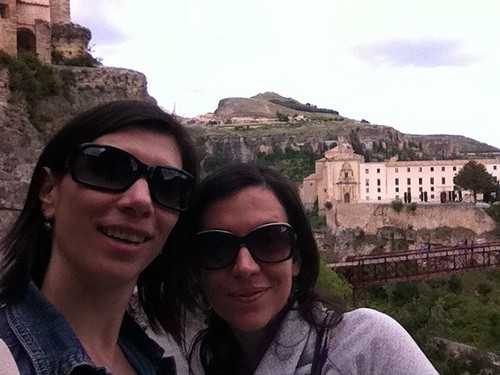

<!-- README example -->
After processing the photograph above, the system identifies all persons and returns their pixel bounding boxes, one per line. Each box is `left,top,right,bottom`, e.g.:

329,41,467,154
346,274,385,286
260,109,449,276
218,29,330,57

183,164,440,375
0,100,198,375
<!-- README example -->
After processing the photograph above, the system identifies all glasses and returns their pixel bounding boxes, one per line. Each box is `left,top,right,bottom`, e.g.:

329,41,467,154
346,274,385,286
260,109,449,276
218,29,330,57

189,222,299,271
61,143,198,212
